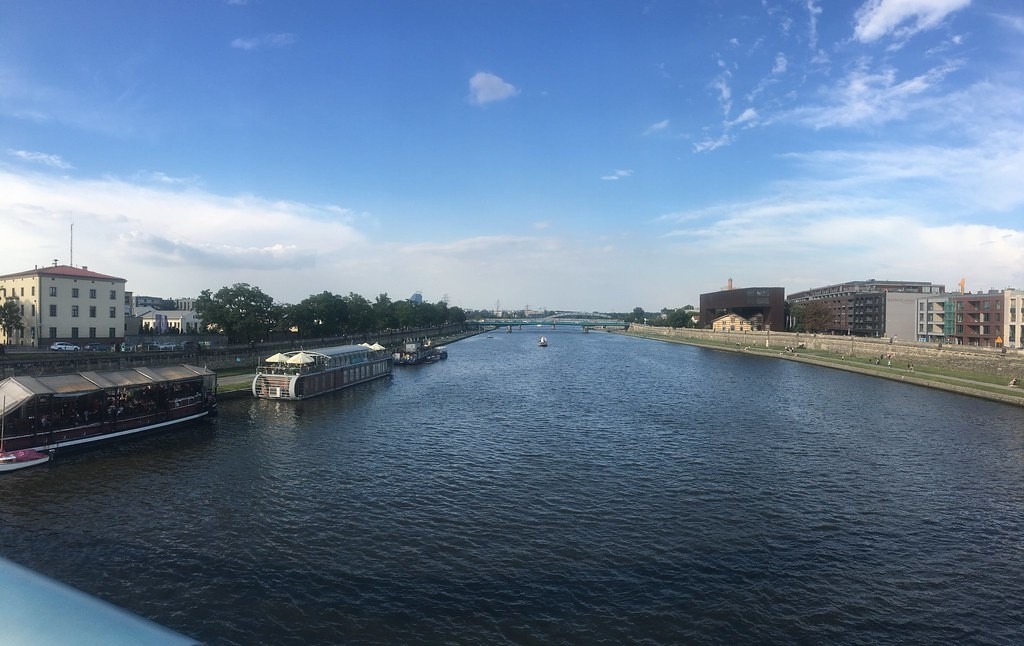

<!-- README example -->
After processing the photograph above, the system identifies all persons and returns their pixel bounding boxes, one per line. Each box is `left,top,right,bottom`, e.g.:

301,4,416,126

270,362,312,375
9,382,202,430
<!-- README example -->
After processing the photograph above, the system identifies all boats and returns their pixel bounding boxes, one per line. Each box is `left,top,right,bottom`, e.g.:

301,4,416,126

0,363,219,452
538,337,547,346
391,337,451,365
0,450,51,472
251,340,395,401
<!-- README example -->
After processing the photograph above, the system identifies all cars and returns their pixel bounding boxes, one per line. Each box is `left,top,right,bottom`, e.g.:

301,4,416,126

135,340,202,350
83,342,110,351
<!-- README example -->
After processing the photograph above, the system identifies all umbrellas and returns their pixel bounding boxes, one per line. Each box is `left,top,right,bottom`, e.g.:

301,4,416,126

362,342,371,348
369,342,385,354
286,352,314,373
265,353,289,373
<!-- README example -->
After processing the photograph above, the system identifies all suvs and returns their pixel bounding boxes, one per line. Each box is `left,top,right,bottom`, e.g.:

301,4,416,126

50,342,80,351
